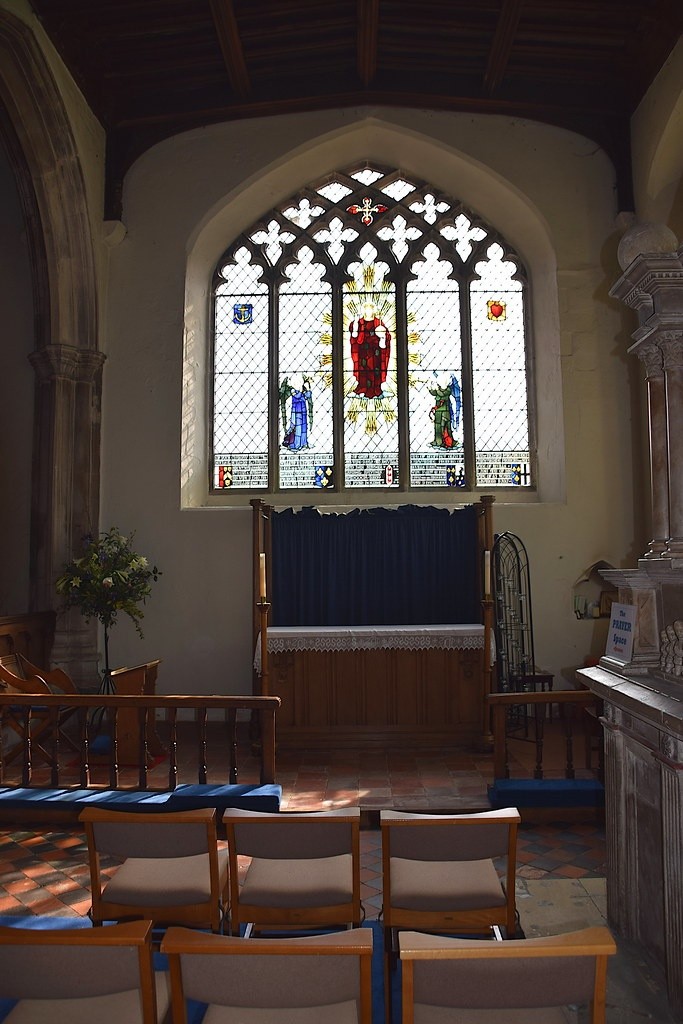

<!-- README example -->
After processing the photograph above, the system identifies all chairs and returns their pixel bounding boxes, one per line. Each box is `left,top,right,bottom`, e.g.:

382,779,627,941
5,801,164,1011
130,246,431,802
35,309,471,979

0,654,82,773
1,801,620,1024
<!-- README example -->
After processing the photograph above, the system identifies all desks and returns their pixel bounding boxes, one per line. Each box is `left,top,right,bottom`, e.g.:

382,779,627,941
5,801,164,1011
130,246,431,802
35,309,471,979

519,666,555,723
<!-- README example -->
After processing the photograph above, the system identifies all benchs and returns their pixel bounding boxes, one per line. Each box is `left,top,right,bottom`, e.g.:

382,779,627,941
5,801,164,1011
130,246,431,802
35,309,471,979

489,778,608,823
1,785,286,825
